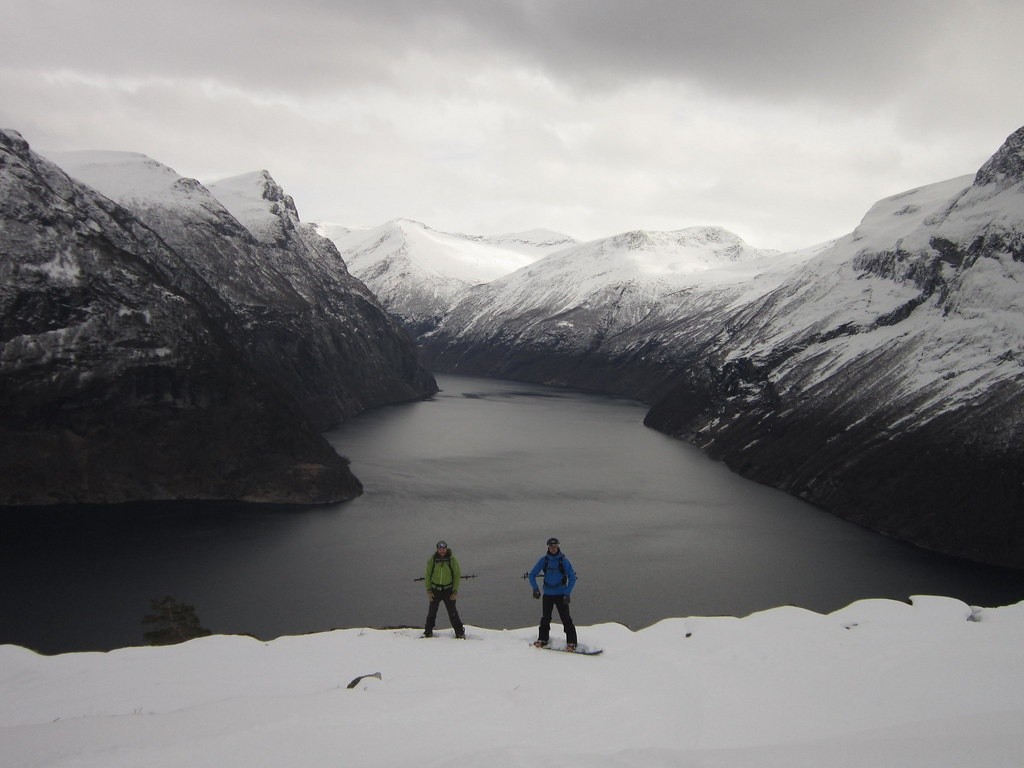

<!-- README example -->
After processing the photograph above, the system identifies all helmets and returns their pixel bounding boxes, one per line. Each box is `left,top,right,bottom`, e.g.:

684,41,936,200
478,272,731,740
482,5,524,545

547,538,560,545
436,540,447,548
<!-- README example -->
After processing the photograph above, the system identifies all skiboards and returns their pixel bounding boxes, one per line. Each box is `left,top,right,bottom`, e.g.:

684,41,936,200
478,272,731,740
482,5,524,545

414,632,480,640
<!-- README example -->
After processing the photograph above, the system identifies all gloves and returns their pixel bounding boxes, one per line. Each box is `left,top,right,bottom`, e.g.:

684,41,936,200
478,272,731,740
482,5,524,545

429,592,435,601
564,595,570,605
450,591,457,601
533,589,541,599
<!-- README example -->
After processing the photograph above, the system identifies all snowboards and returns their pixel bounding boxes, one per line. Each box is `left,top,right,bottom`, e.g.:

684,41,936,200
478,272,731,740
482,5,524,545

534,639,603,655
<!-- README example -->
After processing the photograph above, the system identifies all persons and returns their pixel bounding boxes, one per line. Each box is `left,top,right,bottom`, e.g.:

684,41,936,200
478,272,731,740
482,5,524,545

418,541,466,639
529,538,578,653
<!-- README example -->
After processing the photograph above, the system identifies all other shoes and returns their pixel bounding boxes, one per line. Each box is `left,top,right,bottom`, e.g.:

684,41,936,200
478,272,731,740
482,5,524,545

420,629,432,638
566,643,576,652
534,640,547,648
456,630,464,639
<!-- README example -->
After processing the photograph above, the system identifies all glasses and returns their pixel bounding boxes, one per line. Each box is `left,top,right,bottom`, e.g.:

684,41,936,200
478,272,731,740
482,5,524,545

549,545,558,549
437,543,446,548
